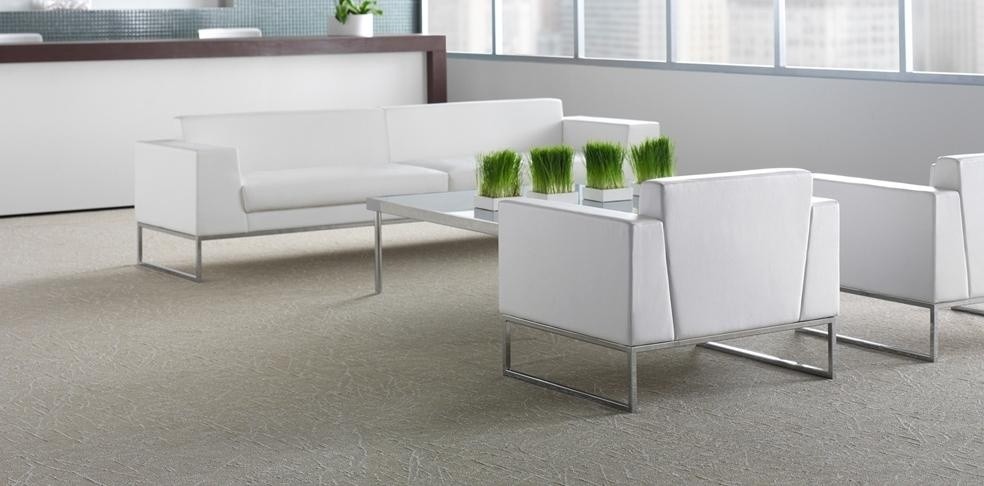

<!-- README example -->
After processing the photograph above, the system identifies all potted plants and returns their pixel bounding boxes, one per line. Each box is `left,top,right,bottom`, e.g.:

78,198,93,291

627,138,673,196
580,141,634,203
531,148,579,206
326,0,382,37
473,149,522,211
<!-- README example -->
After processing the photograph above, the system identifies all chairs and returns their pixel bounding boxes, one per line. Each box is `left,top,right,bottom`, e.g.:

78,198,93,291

198,26,264,38
1,31,44,42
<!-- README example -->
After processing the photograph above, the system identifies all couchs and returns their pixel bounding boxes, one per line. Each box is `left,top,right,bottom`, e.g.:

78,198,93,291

795,151,983,365
132,97,662,283
498,166,843,415
